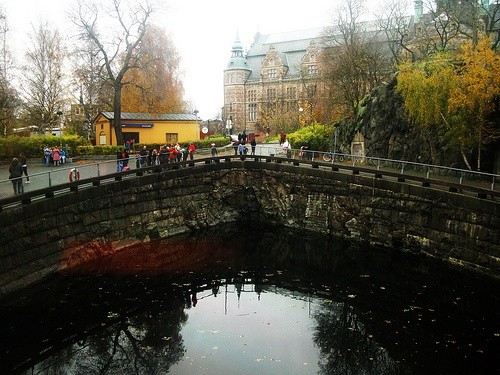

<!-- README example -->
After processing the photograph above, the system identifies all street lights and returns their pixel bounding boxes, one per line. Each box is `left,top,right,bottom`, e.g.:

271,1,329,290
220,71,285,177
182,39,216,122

299,108,304,129
333,122,339,162
56,111,63,147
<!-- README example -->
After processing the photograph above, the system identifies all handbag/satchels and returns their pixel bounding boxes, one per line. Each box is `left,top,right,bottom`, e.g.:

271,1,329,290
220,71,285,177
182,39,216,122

283,147,288,151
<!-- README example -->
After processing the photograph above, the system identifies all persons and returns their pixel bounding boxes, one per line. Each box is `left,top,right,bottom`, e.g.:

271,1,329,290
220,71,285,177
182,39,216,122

19,152,30,184
117,147,130,176
251,139,256,152
300,142,312,160
135,142,195,168
9,158,23,194
126,138,135,154
283,138,291,159
210,143,217,162
42,144,70,167
280,131,284,144
233,131,248,154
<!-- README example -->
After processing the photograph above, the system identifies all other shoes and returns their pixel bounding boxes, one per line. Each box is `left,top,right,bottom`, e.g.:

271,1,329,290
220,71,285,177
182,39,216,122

24,181,30,183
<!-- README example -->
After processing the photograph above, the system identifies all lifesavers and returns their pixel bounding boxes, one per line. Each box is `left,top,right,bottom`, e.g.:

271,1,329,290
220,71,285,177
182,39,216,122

69,168,80,182
